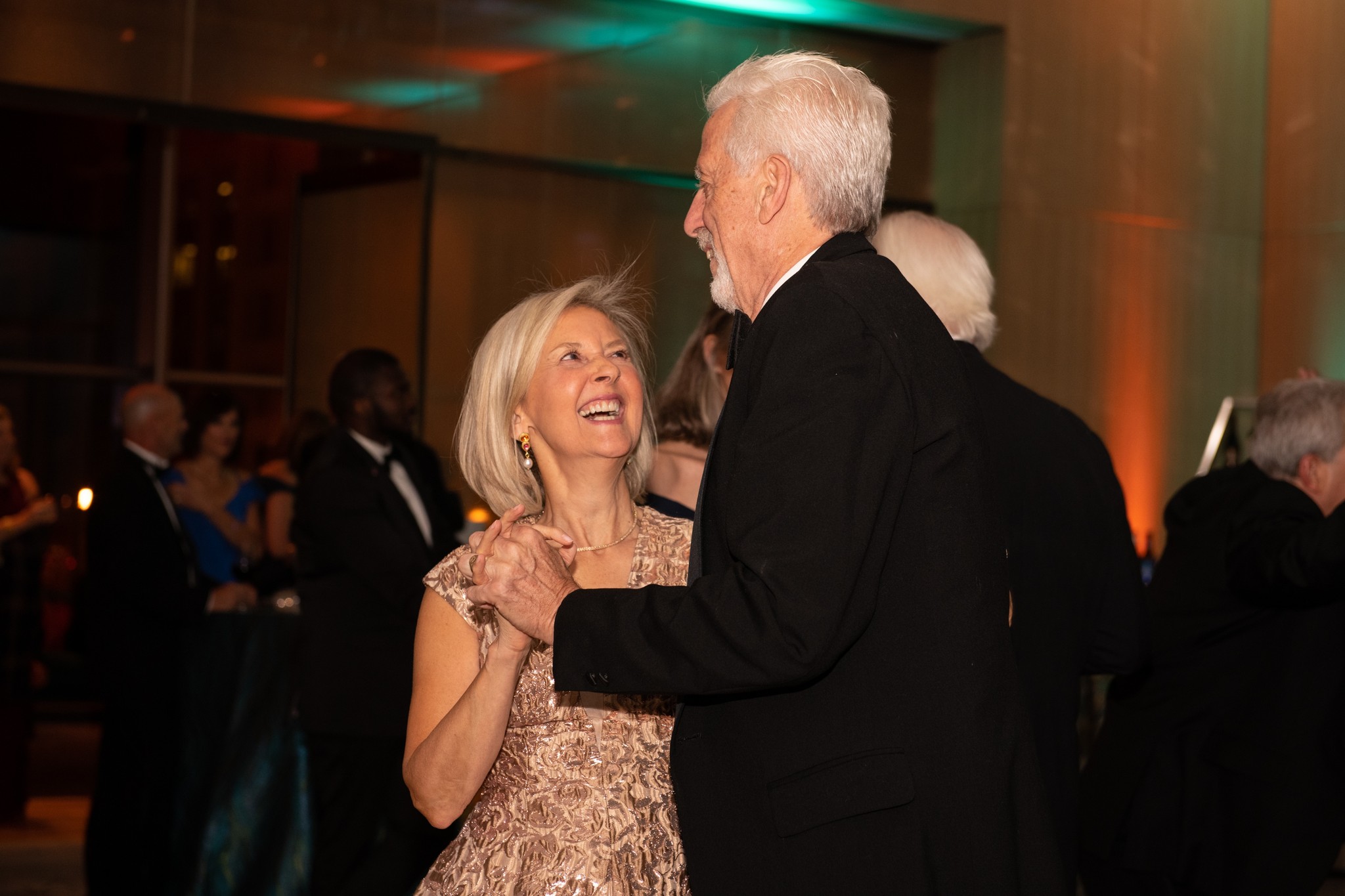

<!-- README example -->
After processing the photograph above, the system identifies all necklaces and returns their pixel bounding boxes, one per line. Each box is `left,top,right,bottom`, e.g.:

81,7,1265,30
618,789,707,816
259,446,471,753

528,508,638,556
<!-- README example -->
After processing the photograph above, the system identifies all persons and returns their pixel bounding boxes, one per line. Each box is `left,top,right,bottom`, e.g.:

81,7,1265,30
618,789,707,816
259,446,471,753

400,250,695,896
1148,372,1345,894
0,208,1149,896
461,43,1037,896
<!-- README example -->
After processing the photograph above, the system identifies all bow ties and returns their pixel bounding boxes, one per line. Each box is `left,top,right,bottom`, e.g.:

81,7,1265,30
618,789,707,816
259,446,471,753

726,308,750,370
382,448,399,475
